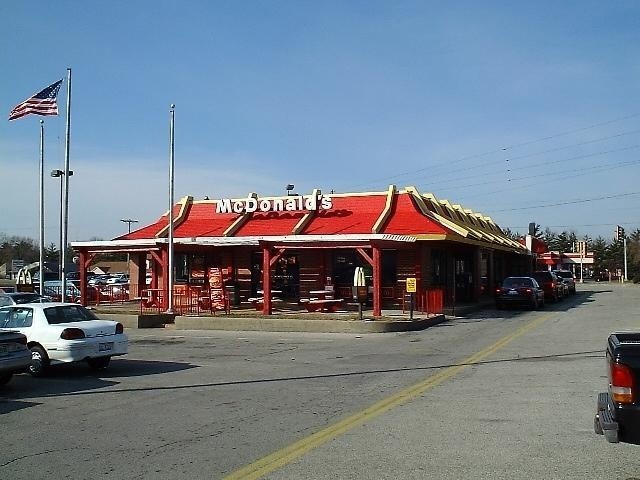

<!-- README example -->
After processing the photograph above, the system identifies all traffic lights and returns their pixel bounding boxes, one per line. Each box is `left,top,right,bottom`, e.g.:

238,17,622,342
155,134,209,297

574,241,589,253
617,225,624,241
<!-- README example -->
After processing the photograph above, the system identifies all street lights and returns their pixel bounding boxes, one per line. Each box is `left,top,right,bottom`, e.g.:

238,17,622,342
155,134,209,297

120,219,138,234
51,169,74,280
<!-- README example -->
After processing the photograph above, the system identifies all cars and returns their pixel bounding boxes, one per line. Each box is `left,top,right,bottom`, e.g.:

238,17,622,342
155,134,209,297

0,271,153,305
480,276,488,295
593,273,609,282
1,301,129,377
495,270,576,310
1,330,32,385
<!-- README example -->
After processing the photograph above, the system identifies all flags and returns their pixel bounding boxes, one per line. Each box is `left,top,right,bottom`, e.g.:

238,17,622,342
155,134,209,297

8,79,63,119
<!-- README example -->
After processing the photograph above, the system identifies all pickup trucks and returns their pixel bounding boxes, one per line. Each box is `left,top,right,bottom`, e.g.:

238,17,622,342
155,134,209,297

594,329,640,446
